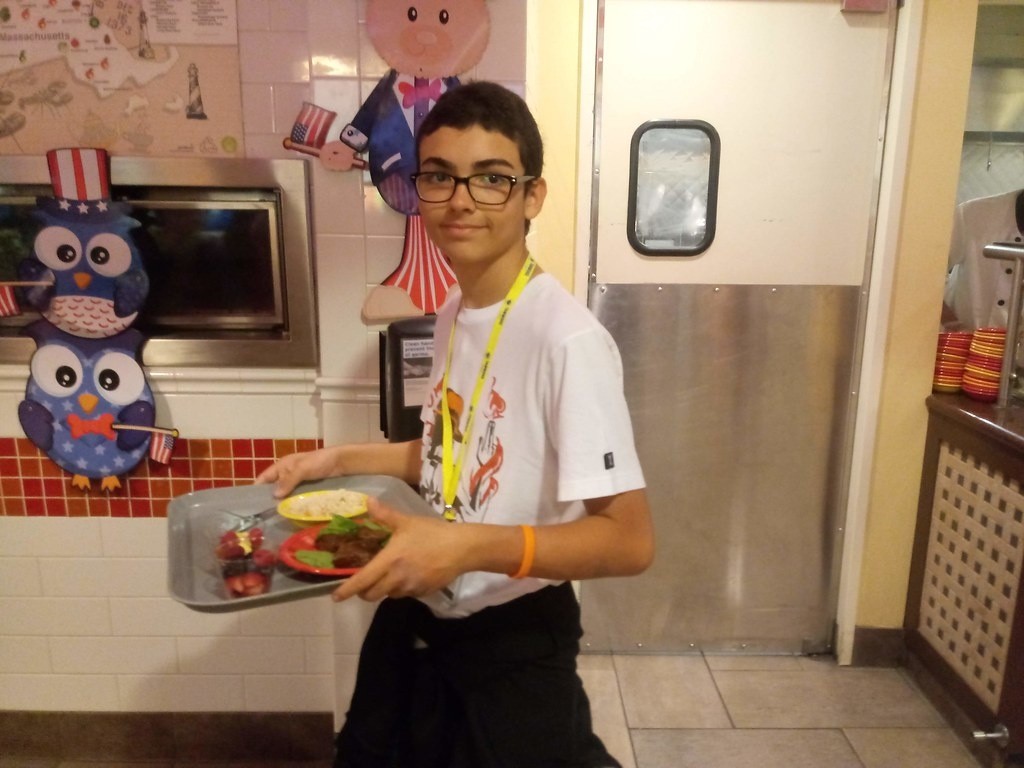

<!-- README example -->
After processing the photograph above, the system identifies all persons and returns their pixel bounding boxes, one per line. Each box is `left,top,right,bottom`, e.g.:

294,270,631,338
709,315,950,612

939,188,1024,374
254,79,655,768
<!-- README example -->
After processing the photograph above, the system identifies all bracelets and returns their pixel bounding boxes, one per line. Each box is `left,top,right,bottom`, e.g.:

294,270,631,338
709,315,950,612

510,524,536,580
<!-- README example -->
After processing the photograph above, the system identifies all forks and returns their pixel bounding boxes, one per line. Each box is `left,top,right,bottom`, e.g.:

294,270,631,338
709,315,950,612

218,508,278,522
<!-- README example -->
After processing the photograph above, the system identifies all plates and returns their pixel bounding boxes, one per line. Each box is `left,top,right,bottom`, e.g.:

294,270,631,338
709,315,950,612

278,519,391,575
934,326,1007,400
275,490,371,525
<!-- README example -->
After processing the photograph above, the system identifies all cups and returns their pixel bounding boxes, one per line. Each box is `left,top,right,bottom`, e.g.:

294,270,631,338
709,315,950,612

215,517,264,551
214,537,274,594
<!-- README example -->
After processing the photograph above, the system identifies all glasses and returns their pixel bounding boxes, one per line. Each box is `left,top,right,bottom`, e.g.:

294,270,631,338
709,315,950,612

409,171,536,207
